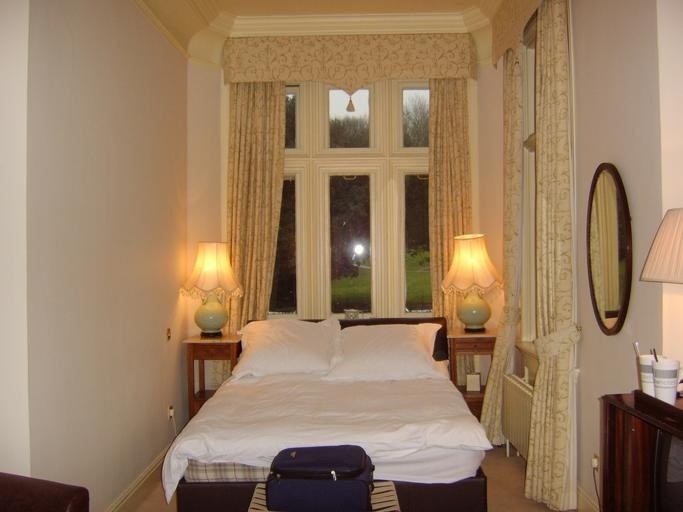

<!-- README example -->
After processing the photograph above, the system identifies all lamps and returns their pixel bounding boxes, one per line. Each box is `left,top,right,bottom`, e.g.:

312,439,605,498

441,233,498,332
640,207,683,284
180,240,244,337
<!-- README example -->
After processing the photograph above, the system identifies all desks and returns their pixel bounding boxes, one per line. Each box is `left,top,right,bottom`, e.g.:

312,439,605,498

597,390,683,511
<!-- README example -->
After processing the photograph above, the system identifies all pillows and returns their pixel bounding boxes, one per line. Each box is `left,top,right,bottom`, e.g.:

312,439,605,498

327,322,445,379
231,318,341,376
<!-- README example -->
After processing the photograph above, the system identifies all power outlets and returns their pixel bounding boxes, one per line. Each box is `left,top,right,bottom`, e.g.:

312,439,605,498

169,405,173,419
593,454,600,471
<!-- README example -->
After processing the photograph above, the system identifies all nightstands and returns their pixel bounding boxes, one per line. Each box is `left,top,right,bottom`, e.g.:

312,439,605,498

447,333,496,402
182,335,242,418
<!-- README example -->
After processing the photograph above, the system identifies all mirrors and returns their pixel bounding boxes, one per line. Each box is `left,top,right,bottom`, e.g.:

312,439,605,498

586,162,632,334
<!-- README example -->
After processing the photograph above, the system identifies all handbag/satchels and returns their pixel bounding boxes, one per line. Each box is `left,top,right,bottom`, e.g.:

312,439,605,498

266,445,374,512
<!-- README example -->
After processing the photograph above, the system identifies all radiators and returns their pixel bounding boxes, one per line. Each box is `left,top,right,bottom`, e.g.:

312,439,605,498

502,374,534,460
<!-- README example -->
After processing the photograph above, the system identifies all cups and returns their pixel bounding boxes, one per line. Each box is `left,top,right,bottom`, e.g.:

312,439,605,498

637,355,678,408
344,309,360,320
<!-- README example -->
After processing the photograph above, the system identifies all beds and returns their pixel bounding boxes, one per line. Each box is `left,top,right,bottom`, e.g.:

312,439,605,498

161,317,494,512
